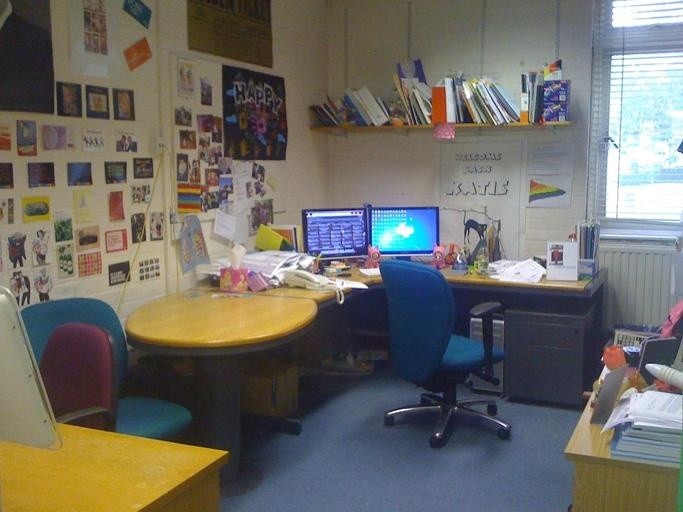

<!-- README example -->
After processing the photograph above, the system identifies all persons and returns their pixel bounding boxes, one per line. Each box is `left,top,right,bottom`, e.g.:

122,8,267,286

32,230,49,265
7,232,26,268
9,270,30,305
433,243,445,269
34,268,50,302
116,134,137,152
365,244,381,267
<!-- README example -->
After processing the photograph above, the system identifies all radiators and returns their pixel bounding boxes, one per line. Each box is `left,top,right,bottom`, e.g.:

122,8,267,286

596,244,679,339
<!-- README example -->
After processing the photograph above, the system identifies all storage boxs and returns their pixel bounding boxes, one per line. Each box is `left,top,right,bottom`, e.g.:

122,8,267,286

238,357,300,418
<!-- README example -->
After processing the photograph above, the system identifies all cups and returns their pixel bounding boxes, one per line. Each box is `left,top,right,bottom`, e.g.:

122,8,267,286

603,346,625,371
623,346,640,368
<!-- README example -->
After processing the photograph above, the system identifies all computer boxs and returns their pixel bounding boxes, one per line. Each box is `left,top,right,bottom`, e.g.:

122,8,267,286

470,315,504,396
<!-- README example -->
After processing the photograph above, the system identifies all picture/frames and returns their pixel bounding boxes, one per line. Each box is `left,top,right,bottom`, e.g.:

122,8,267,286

525,175,573,209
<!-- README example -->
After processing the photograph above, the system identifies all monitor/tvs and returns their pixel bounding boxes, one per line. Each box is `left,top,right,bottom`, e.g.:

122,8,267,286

302,207,369,264
369,206,440,261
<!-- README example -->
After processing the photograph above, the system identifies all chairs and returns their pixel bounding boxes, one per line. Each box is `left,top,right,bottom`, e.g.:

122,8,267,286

38,322,117,432
20,298,198,446
378,257,512,447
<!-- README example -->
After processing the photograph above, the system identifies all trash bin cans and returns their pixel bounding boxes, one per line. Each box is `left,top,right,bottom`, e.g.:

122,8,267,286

138,355,205,414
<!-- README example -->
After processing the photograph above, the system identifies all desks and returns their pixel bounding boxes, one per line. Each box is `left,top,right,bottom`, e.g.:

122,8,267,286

563,331,682,512
203,257,609,407
0,423,231,512
124,288,318,497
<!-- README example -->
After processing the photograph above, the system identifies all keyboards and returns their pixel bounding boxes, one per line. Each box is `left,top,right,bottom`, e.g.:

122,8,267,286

360,267,380,276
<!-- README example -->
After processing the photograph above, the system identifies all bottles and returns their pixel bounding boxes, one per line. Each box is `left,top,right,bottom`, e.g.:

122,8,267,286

444,243,466,271
476,240,489,278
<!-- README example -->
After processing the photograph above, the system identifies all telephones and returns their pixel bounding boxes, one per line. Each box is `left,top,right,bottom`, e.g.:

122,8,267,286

285,270,328,288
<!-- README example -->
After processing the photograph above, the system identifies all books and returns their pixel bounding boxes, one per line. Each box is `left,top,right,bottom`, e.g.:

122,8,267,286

309,71,538,127
610,390,683,462
613,330,653,347
